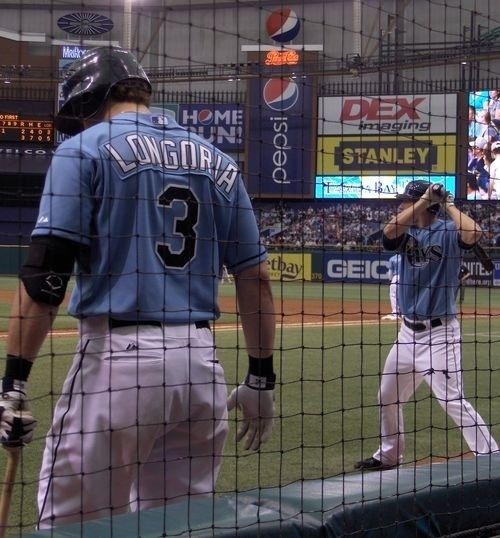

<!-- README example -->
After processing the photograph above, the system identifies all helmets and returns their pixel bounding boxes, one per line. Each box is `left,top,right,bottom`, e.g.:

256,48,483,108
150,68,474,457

54,45,152,136
395,180,440,215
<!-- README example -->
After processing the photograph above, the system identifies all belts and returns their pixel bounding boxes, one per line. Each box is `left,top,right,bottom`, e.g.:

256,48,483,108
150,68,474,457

404,317,442,331
109,318,210,330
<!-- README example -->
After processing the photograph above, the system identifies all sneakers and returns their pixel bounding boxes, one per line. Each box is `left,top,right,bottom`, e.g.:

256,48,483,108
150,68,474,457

354,457,382,469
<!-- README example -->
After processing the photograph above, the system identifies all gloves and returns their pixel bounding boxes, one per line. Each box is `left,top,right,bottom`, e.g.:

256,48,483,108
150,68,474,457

0,353,38,447
227,354,276,450
420,183,454,211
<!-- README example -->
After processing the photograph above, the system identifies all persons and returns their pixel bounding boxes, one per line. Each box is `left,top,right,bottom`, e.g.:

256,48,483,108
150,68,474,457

356,180,499,470
468,90,500,200
0,46,276,531
256,201,500,252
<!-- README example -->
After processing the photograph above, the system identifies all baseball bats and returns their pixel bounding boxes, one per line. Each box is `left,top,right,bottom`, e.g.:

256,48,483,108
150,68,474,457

432,184,495,273
1,400,26,537
